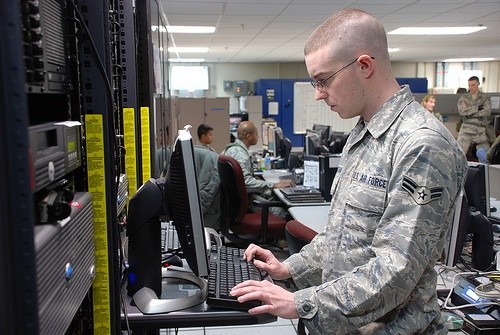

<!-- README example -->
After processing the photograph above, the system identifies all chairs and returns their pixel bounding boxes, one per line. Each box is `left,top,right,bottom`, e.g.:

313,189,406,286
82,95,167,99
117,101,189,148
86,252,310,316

217,156,290,252
285,220,319,335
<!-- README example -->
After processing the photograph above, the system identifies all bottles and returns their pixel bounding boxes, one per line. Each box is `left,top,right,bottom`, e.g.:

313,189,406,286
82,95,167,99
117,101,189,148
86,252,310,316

265,153,271,170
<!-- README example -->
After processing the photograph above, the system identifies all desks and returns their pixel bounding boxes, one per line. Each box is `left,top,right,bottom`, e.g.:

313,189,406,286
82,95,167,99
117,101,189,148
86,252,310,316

260,169,330,209
286,205,330,234
119,266,278,335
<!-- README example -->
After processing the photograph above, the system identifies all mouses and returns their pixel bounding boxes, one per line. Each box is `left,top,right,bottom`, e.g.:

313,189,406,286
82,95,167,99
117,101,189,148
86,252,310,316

160,254,183,267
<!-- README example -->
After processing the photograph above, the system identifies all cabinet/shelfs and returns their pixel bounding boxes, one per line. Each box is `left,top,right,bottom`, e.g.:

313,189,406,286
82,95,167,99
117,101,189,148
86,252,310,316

170,97,230,154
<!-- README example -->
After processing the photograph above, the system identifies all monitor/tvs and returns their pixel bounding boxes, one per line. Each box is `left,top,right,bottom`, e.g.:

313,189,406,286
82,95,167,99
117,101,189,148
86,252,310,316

304,123,331,157
445,186,495,271
302,155,327,194
125,131,210,315
463,161,490,218
260,122,290,163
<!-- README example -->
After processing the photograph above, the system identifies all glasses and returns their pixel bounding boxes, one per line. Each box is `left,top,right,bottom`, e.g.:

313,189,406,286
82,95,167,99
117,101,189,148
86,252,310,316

310,56,375,91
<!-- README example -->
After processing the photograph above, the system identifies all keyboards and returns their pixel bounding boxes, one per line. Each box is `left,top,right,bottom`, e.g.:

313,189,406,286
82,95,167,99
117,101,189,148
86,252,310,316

159,223,185,258
279,174,301,184
204,243,263,312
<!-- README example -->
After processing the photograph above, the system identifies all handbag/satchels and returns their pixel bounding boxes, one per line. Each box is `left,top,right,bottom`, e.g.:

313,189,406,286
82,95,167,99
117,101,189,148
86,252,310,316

485,124,497,146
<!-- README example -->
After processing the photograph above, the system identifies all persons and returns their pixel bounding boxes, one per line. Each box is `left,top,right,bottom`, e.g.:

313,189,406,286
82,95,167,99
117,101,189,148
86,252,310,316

194,124,216,152
457,76,492,164
230,9,470,335
421,95,443,123
220,121,296,218
